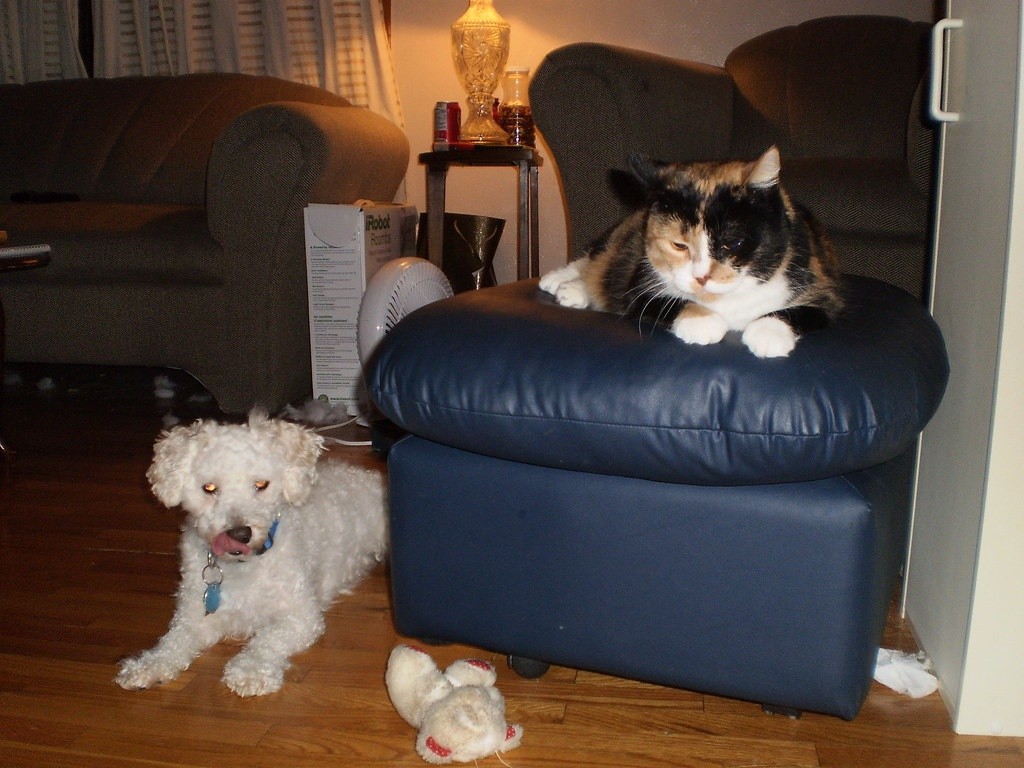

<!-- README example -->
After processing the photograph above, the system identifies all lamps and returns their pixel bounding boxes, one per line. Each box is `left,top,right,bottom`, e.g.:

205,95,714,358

450,0,513,145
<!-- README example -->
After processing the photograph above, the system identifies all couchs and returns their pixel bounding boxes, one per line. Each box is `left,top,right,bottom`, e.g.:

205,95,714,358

361,276,948,723
0,71,410,417
526,11,942,307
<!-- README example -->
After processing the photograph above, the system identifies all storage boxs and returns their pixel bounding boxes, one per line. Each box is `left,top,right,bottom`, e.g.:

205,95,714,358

302,199,440,430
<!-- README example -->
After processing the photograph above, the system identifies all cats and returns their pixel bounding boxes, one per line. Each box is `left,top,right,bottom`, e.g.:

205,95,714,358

539,145,847,360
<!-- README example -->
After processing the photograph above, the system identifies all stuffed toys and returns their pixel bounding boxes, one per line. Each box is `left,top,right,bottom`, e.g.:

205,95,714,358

383,644,524,766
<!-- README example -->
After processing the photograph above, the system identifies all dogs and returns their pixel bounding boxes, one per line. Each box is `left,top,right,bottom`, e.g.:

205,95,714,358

112,403,390,697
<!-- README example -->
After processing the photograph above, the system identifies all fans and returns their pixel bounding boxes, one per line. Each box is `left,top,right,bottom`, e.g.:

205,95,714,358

355,256,455,368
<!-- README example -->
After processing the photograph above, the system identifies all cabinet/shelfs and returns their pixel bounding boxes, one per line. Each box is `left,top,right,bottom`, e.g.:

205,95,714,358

899,0,1024,741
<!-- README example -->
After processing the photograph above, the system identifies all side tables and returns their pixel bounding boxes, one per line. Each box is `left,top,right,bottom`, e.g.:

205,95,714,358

416,143,543,282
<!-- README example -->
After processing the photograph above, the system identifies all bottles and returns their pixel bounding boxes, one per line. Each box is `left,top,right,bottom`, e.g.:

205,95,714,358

496,63,536,150
